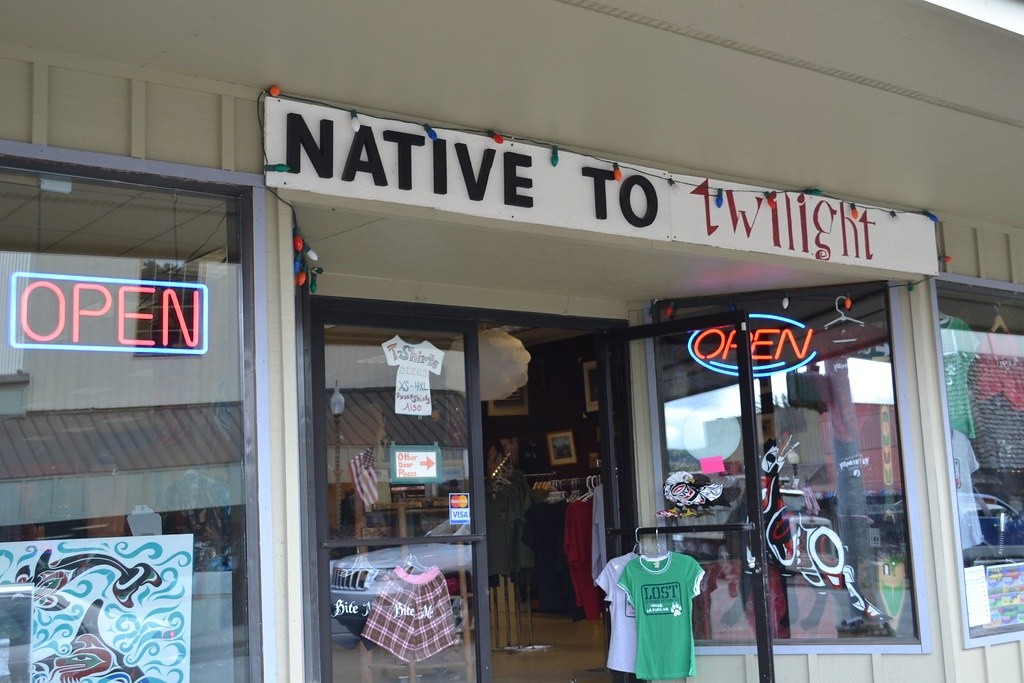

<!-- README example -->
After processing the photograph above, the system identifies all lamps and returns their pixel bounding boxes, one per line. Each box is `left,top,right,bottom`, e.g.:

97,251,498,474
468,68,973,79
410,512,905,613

443,320,532,402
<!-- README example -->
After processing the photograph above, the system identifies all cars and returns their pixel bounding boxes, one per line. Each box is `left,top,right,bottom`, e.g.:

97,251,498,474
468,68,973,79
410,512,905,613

0,585,144,682
873,494,1024,562
333,513,478,649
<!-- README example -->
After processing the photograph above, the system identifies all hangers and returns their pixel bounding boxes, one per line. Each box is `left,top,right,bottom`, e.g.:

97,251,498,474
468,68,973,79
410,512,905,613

383,537,444,581
937,305,967,327
484,454,605,511
168,459,231,493
331,541,391,578
976,300,1023,350
978,380,1023,411
607,527,697,566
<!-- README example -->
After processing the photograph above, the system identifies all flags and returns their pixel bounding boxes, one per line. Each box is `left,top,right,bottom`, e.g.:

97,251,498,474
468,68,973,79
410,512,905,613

349,443,380,506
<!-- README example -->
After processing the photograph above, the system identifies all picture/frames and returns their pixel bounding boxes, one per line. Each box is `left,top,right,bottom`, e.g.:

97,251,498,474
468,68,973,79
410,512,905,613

487,381,529,418
389,442,442,485
589,452,599,467
546,429,577,465
597,426,601,442
580,355,599,415
